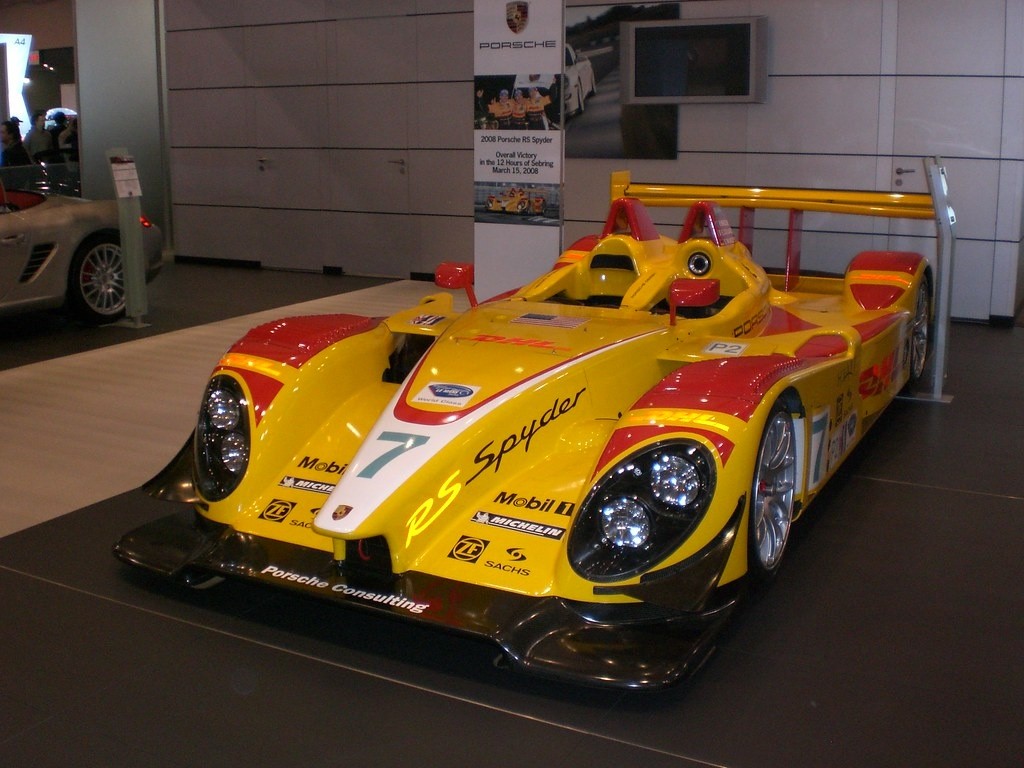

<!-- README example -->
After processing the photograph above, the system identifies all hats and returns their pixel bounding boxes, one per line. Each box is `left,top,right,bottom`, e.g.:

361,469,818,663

11,117,23,122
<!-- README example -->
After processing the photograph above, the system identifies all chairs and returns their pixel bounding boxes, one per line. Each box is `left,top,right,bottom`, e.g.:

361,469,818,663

651,239,748,313
568,235,644,308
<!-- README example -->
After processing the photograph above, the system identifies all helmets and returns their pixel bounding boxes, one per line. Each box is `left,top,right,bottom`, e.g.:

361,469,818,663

529,87,537,91
499,89,509,96
515,89,522,94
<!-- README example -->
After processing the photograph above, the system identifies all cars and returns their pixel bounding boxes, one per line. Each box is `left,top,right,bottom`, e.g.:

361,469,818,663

512,44,597,120
0,164,164,326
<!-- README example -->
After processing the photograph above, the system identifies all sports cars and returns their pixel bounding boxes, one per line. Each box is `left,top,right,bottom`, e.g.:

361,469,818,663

112,195,932,690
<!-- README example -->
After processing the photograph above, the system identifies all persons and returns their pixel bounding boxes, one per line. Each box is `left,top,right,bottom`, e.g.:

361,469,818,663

475,87,553,130
1,111,79,187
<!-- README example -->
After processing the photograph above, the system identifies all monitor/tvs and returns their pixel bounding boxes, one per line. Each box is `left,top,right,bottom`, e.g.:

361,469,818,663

619,15,769,104
